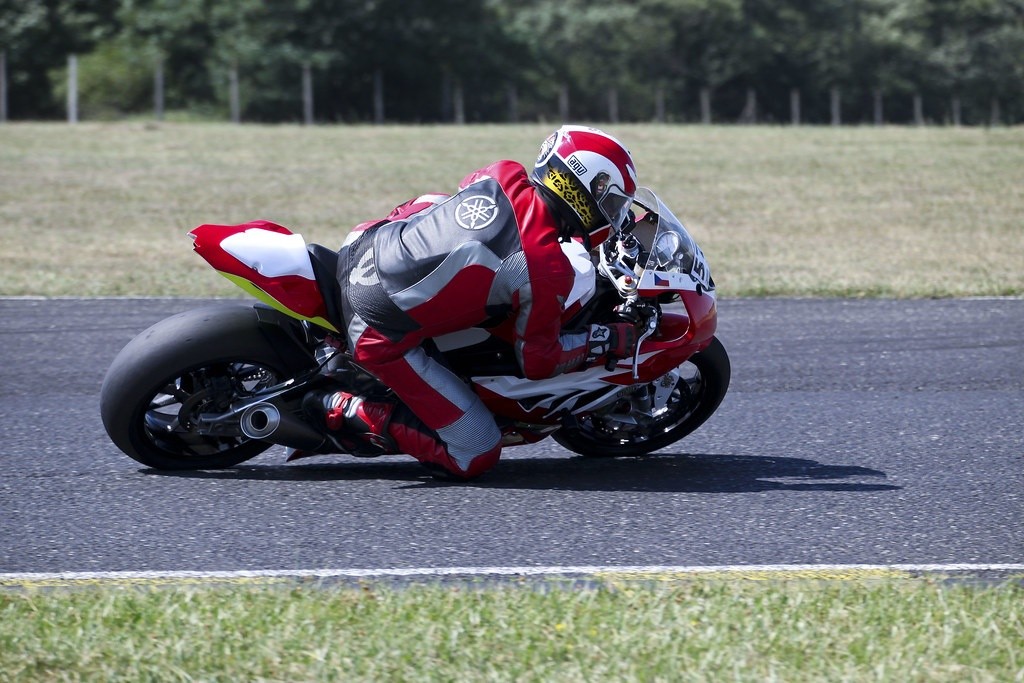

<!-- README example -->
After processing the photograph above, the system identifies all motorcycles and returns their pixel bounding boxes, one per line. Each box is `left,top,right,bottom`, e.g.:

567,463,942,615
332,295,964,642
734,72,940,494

99,184,731,475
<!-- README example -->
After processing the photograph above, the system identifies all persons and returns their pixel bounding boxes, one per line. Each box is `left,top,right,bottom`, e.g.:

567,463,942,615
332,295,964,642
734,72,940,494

303,124,638,478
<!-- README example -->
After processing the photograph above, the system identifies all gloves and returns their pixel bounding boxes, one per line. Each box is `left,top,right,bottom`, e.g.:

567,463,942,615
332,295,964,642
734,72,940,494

582,322,637,371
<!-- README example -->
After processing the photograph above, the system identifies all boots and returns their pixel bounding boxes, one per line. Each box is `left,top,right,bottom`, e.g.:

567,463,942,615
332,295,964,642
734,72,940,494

301,387,400,457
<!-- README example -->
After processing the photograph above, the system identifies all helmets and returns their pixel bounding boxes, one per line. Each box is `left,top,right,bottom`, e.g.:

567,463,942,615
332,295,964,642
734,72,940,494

530,123,636,253
